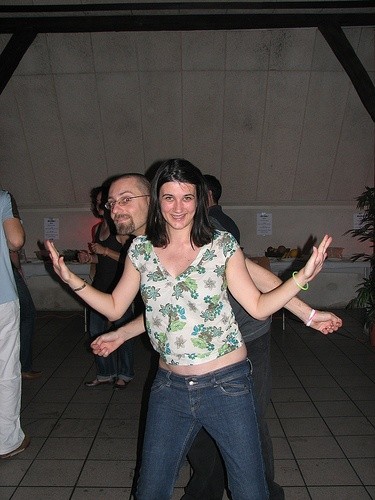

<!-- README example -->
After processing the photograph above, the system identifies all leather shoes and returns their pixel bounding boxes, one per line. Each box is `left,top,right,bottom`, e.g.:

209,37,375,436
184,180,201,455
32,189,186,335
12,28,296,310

112,379,131,390
85,378,113,387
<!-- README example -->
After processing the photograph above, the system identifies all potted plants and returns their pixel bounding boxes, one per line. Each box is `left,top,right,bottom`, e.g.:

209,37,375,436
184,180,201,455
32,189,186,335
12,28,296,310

341,186,375,349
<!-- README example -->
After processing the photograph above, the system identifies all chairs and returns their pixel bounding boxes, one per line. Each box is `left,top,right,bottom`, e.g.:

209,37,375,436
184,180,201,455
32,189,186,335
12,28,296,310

250,256,286,331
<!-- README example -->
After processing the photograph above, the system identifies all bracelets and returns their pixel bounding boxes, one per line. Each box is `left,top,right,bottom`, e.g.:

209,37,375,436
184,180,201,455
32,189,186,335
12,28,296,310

292,271,308,290
305,309,316,326
73,280,86,292
103,247,107,257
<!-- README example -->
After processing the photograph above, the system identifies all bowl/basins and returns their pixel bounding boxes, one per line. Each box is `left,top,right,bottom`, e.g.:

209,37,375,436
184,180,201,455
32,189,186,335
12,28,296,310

325,247,344,258
34,250,50,260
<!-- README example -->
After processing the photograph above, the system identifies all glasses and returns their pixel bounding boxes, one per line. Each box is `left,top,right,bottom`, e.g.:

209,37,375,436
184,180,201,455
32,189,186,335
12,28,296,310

105,195,151,209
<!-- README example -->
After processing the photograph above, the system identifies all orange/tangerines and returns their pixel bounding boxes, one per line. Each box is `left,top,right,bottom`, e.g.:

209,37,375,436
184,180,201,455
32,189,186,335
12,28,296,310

290,249,298,257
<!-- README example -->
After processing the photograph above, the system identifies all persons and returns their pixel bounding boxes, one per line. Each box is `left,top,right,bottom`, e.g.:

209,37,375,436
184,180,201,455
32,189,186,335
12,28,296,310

0,188,31,459
9,248,42,380
76,174,343,500
44,158,332,500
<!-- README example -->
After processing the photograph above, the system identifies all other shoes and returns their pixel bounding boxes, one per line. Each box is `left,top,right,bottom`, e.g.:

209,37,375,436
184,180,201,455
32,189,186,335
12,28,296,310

22,371,42,379
0,434,31,458
